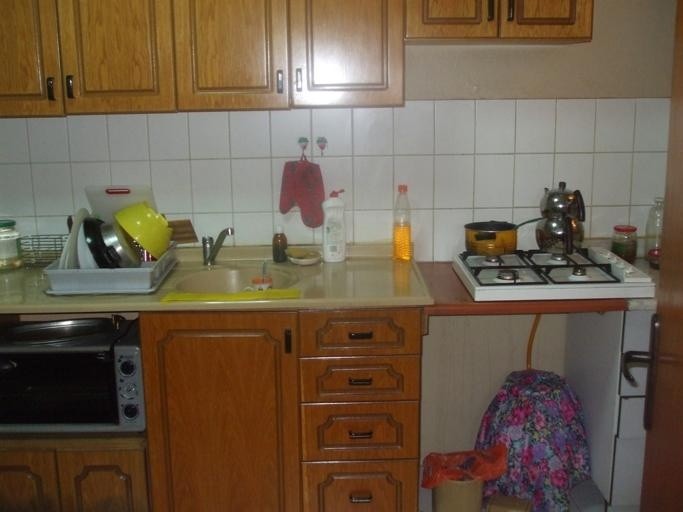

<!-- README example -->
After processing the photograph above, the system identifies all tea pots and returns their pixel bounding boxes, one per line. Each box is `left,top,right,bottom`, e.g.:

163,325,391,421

534,178,588,254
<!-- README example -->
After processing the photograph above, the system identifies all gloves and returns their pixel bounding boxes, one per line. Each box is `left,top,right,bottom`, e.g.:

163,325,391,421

279,159,325,228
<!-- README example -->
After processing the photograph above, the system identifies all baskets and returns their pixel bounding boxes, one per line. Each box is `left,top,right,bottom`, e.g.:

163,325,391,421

16,234,68,267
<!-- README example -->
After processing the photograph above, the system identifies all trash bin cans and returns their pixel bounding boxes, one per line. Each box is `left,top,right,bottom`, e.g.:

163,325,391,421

433,453,486,511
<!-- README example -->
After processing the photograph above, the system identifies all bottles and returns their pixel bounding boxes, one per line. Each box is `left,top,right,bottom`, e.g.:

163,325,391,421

322,259,349,302
393,260,412,298
321,188,346,265
272,223,285,262
646,197,663,267
0,217,24,271
391,183,411,261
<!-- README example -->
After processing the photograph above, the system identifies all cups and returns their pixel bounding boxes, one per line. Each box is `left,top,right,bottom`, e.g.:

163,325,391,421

244,276,271,292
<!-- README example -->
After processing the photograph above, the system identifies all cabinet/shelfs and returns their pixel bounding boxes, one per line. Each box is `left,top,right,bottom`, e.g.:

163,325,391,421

0,0,595,116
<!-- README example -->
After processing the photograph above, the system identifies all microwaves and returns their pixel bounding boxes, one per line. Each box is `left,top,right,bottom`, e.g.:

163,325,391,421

0,318,147,436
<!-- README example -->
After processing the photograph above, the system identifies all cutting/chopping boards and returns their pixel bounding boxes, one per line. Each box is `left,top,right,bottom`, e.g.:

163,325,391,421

82,183,157,222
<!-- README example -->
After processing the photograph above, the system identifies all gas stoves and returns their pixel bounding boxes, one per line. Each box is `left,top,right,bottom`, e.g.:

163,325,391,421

451,244,654,302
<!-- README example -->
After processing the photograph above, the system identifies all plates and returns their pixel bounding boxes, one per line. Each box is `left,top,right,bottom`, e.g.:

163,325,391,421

289,254,319,267
58,205,171,272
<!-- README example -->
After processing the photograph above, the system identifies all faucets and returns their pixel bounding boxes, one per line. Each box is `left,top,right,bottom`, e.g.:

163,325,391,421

203,228,233,264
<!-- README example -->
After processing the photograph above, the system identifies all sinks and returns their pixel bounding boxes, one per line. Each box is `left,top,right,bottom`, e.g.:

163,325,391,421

177,268,291,293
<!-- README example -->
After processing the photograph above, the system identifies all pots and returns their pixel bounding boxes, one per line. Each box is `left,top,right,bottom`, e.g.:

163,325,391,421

464,214,547,255
